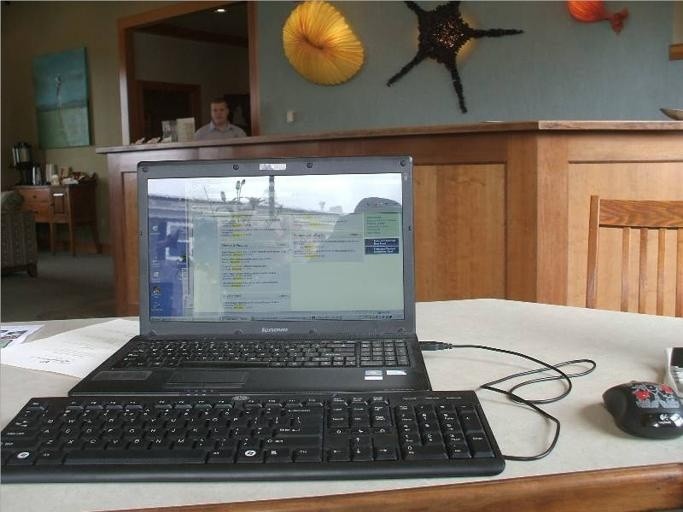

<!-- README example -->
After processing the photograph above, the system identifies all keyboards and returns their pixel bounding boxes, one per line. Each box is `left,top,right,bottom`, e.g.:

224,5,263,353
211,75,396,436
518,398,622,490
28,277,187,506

0,391,505,484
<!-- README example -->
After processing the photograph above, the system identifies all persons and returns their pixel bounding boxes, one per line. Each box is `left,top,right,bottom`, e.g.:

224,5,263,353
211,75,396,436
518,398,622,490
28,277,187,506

195,97,248,139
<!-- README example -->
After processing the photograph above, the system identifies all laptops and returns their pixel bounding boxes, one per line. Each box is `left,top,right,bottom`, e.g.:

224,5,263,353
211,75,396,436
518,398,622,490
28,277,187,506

68,155,433,398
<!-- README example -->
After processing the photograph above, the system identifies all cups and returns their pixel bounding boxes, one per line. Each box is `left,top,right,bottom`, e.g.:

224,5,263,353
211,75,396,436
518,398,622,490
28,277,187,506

59,167,71,177
159,120,177,142
174,117,194,142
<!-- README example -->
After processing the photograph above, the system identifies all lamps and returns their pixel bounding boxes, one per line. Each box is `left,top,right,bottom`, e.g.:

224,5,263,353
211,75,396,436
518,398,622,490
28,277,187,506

8,142,38,184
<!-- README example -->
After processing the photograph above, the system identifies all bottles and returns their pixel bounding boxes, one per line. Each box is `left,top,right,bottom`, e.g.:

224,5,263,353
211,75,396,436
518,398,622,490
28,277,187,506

31,160,42,186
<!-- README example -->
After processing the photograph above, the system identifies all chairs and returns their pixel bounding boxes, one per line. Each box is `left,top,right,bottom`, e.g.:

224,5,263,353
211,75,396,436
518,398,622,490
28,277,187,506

586,195,683,318
1,191,38,276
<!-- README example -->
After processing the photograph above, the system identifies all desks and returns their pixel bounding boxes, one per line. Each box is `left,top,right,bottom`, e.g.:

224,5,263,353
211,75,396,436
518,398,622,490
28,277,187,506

0,298,683,512
8,183,102,256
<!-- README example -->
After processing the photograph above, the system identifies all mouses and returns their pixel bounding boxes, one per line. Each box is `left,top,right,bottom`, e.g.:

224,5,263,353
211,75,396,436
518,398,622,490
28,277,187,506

603,380,683,439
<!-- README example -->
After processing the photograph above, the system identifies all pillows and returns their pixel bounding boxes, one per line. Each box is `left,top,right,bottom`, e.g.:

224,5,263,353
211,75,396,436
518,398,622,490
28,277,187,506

1,191,23,210
